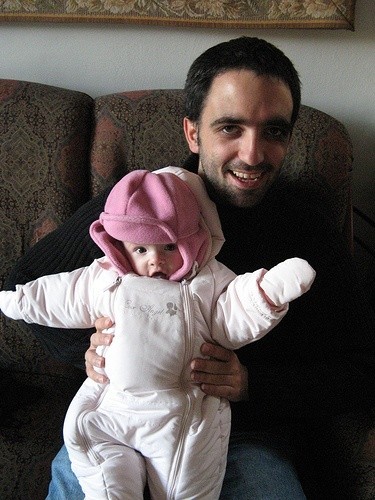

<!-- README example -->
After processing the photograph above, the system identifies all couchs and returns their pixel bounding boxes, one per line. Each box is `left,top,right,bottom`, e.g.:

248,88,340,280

0,79,374,499
